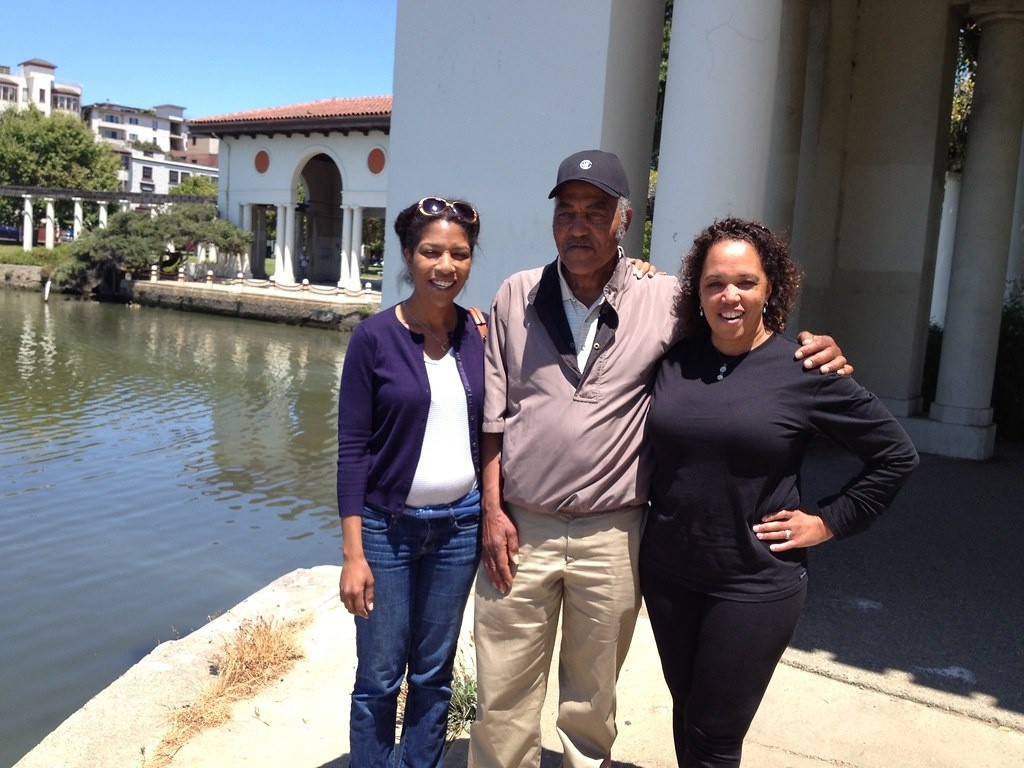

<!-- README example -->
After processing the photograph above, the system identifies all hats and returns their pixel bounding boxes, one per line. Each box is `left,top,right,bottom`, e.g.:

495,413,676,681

548,150,630,200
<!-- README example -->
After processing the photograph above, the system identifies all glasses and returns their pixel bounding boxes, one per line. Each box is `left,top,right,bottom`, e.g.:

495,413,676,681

414,196,479,225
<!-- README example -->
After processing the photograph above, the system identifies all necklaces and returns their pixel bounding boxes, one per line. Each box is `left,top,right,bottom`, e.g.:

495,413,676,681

711,327,766,380
404,300,457,351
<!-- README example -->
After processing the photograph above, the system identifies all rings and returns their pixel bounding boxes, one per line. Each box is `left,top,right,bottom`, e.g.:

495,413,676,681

785,530,790,539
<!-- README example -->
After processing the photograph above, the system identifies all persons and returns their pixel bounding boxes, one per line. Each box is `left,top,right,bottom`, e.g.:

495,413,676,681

467,148,853,768
336,196,667,768
638,215,921,768
